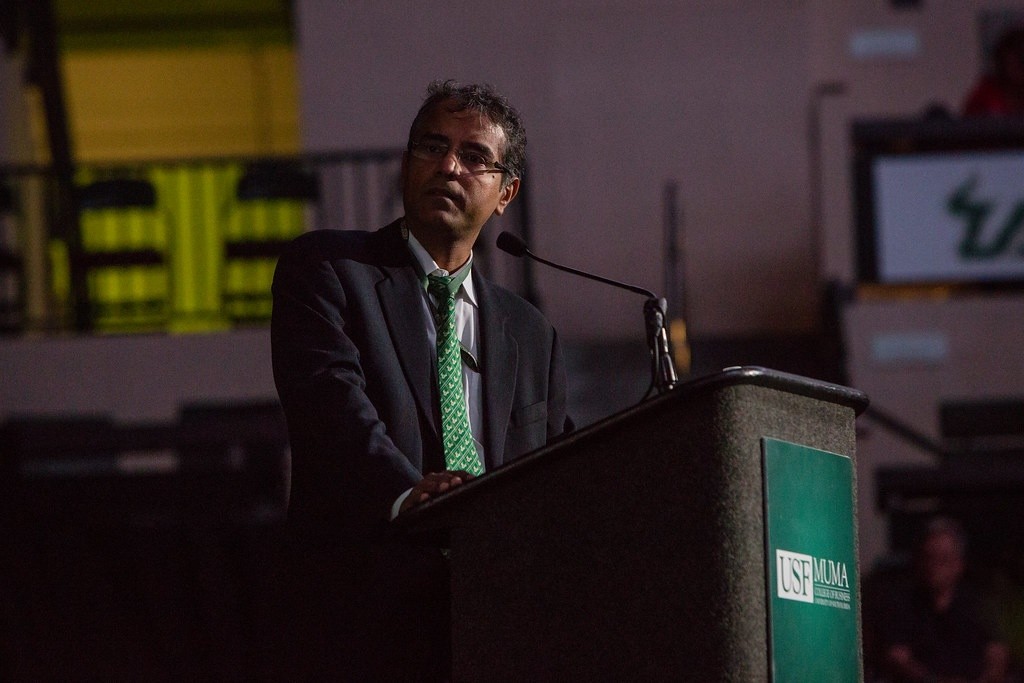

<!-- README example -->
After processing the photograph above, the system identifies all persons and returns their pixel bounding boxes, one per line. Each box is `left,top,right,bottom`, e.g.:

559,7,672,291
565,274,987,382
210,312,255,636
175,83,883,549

270,79,569,525
883,518,1009,683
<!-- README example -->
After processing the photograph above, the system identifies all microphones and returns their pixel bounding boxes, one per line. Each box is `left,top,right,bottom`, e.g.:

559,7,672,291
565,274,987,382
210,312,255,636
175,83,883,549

495,231,678,391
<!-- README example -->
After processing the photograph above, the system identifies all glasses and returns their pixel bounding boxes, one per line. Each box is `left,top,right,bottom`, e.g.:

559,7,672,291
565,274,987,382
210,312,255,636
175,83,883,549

407,137,514,174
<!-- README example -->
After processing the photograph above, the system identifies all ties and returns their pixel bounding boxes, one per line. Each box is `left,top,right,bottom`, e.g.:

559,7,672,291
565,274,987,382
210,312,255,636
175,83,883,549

426,272,486,477
